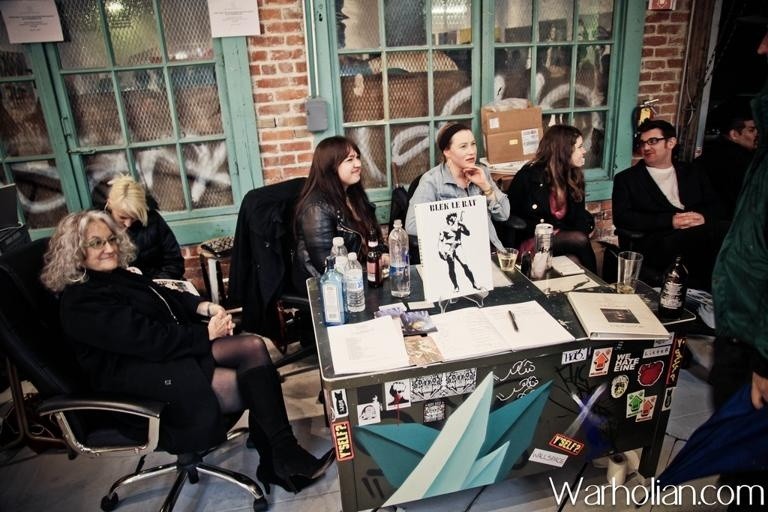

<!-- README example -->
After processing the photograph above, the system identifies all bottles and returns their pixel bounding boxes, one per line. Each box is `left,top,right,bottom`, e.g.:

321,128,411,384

361,231,386,286
521,222,554,278
319,236,366,327
656,253,690,318
385,218,410,298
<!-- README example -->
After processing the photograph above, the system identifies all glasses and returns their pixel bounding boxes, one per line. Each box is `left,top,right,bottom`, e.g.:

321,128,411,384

82,235,122,249
635,138,666,147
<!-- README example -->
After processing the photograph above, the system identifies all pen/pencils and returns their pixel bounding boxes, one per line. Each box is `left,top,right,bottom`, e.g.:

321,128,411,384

509,311,518,330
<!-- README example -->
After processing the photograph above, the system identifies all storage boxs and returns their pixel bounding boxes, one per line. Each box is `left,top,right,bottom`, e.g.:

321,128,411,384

479,95,547,161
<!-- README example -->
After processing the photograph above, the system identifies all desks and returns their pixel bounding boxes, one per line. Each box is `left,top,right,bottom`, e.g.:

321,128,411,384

304,255,702,512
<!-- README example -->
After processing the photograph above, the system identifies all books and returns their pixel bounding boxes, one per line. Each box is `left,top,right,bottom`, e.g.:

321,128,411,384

424,298,575,364
565,292,671,339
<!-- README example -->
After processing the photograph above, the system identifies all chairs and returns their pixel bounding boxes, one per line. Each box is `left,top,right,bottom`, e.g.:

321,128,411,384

0,232,278,512
594,229,647,288
383,170,526,263
220,175,317,380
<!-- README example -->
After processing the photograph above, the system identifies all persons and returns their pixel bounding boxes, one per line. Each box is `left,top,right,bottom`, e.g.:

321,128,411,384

291,136,393,298
707,32,767,511
497,123,598,279
610,118,721,233
102,168,185,283
359,381,410,420
437,209,482,291
40,206,324,495
701,93,761,211
404,120,510,259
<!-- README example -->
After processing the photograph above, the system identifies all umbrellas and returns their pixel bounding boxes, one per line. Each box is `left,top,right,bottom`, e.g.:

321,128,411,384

632,381,768,510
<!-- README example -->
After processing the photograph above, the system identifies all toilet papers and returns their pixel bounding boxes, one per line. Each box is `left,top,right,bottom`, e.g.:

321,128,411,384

605,452,629,488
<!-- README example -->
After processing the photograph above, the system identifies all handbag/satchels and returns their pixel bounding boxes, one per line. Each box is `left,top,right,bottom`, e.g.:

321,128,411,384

278,295,314,330
197,235,236,261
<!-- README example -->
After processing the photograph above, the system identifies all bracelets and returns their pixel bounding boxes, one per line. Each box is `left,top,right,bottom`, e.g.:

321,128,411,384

482,189,492,197
206,300,211,318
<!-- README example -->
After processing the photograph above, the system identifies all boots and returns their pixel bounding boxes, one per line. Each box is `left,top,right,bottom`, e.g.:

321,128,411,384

238,364,335,494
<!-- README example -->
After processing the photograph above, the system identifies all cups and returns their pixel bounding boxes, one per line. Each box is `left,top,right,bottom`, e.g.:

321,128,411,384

615,250,643,297
495,247,518,270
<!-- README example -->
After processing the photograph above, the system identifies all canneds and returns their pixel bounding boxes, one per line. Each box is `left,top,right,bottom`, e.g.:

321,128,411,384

535,223,553,271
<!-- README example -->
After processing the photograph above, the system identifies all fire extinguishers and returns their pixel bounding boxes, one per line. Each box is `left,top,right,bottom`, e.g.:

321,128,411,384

632,99,659,154
